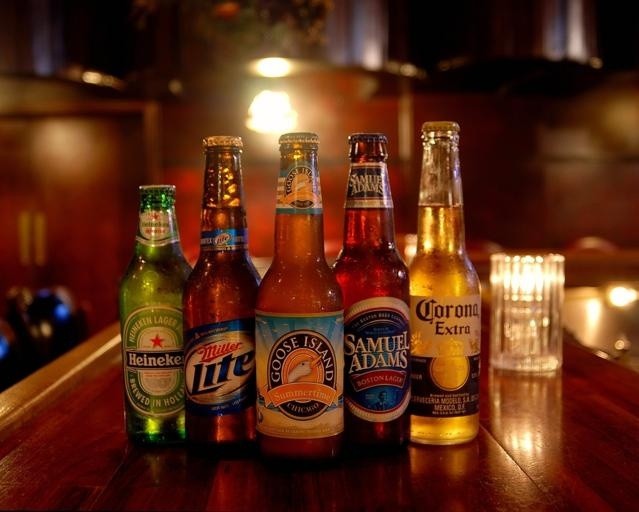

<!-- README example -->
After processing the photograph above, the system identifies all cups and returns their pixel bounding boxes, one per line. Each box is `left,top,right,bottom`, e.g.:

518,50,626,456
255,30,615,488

491,252,565,376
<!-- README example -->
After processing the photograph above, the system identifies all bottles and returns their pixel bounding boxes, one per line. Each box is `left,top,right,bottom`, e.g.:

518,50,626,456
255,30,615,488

329,131,411,443
181,135,262,443
253,131,347,456
122,182,190,450
408,122,483,447
